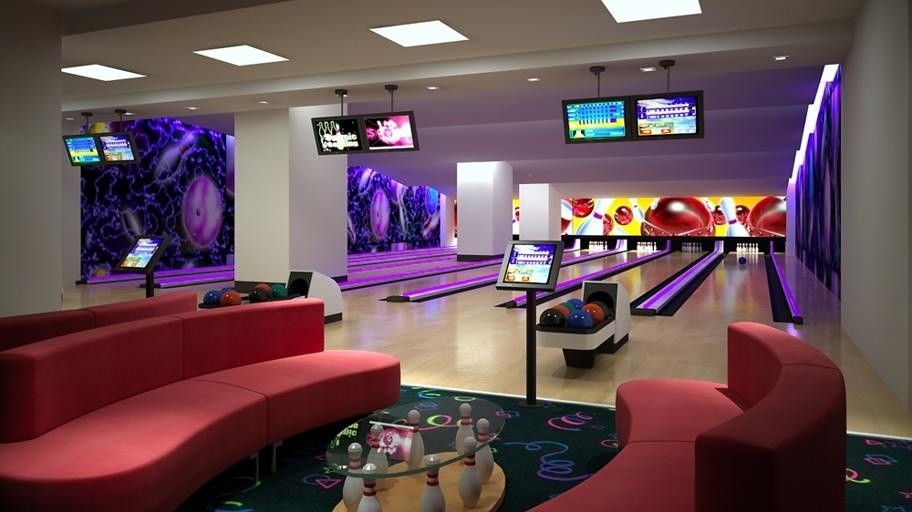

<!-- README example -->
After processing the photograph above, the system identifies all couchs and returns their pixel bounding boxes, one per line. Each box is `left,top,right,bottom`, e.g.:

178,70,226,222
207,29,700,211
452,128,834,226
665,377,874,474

2,288,199,352
520,319,848,512
1,295,404,511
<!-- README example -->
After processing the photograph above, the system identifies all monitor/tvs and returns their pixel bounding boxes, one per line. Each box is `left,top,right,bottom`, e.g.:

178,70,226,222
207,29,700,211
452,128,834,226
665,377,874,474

63,133,104,166
97,131,140,165
495,240,564,292
114,235,168,274
631,90,704,141
311,115,366,155
360,111,419,153
561,96,630,145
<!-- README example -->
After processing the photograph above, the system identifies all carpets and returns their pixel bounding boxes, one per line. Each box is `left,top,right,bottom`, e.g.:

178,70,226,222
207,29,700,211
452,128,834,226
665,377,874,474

175,381,910,511
